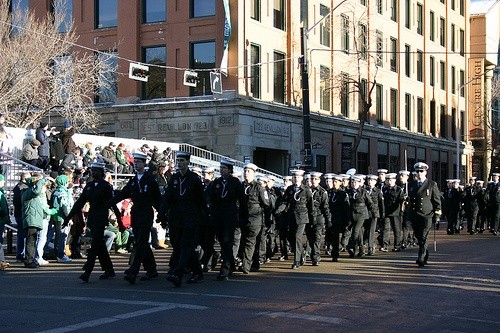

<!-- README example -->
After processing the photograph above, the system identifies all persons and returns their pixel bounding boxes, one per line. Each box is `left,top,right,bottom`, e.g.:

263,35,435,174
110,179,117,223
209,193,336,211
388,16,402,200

274,168,316,270
0,119,500,282
406,161,442,267
61,163,125,282
113,153,167,282
202,159,249,282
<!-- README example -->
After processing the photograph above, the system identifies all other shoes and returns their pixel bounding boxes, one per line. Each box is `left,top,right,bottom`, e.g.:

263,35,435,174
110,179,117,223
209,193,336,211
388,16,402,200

98,272,115,279
57,255,72,262
447,224,500,236
166,241,429,290
38,259,49,266
156,240,168,249
141,271,158,280
71,254,87,259
16,257,25,263
79,274,89,283
115,249,128,254
0,262,10,271
26,262,39,267
124,274,136,284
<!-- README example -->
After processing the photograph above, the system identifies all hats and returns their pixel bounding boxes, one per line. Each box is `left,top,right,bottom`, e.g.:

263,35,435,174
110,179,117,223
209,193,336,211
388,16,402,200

64,120,71,128
110,142,116,146
90,162,105,169
256,162,429,183
143,143,149,148
132,153,147,161
40,121,47,128
446,173,500,184
176,150,191,159
243,162,258,172
220,158,234,169
